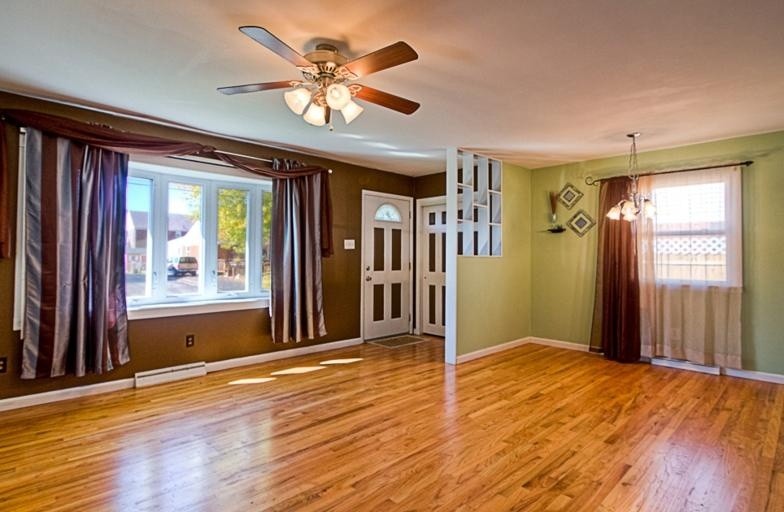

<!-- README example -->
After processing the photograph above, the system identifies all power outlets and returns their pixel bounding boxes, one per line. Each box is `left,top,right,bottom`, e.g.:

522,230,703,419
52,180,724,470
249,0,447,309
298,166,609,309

0,356,6,372
185,334,194,347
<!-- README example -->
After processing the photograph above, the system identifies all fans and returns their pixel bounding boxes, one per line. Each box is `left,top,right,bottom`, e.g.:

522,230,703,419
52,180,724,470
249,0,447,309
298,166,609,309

216,25,420,116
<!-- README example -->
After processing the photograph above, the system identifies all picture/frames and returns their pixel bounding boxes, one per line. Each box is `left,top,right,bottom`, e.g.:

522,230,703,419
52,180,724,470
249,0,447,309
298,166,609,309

554,181,597,238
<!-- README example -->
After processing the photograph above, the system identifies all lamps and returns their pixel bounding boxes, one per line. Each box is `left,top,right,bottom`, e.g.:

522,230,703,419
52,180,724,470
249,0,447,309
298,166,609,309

604,133,658,222
282,83,365,127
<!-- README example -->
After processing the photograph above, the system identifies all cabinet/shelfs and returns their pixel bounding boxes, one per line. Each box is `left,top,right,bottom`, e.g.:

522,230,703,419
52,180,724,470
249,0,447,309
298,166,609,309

446,147,501,256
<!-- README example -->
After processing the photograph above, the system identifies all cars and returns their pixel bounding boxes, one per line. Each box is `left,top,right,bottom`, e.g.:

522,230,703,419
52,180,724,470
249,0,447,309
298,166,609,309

175,256,198,277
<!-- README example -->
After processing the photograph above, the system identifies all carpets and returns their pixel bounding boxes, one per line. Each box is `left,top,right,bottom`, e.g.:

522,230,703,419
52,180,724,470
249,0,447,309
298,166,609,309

366,334,429,350
